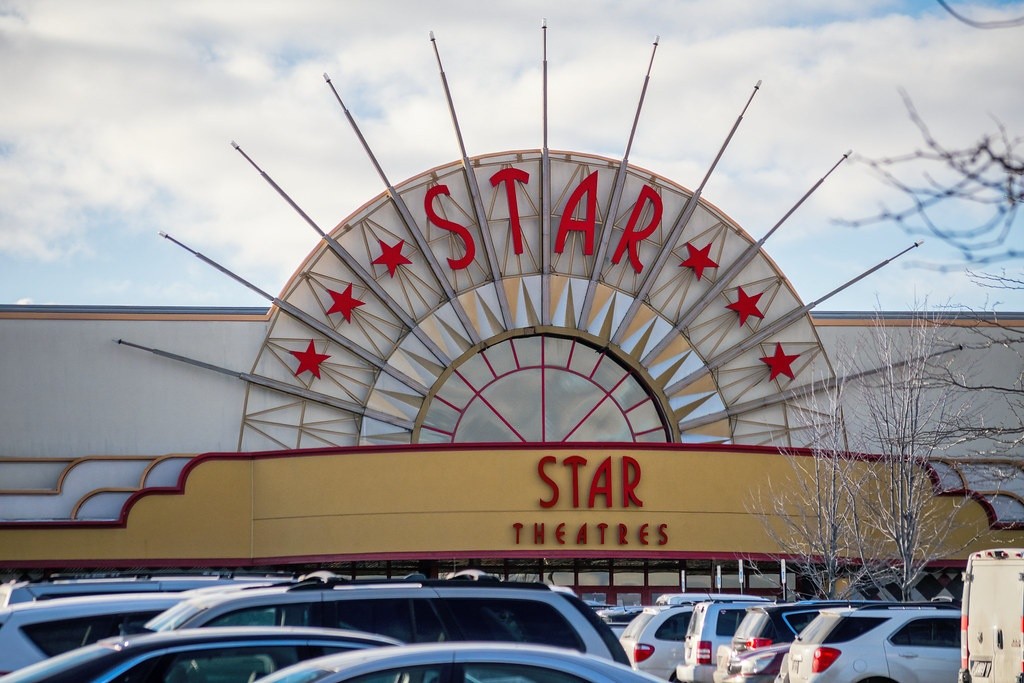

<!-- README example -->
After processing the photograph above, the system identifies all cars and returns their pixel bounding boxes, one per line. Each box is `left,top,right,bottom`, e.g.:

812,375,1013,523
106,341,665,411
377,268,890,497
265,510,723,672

247,640,668,683
4,624,482,683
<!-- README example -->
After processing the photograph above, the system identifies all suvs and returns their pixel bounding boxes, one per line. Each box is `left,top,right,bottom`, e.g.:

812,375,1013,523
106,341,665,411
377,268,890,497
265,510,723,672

0,562,768,671
619,604,699,683
715,599,876,683
773,600,963,682
675,598,780,683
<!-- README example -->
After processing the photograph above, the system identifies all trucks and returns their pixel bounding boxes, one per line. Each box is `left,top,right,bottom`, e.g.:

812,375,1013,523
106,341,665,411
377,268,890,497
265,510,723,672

958,548,1023,683
